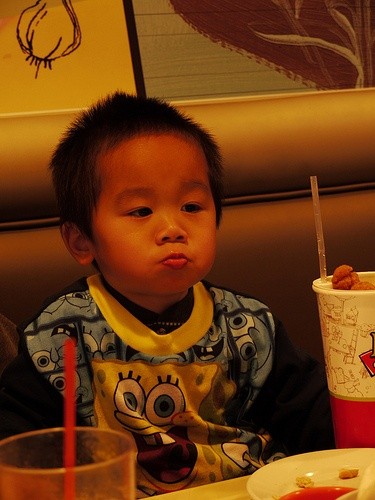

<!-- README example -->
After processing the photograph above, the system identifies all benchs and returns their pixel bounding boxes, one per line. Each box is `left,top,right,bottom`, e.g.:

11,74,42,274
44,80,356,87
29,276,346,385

0,87,375,439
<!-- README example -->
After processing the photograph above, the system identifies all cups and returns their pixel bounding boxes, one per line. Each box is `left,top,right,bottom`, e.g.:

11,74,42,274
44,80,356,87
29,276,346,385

311,271,375,448
0,426,137,500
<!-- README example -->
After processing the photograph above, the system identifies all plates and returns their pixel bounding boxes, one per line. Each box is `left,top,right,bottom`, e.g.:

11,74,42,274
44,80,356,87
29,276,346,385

247,448,375,500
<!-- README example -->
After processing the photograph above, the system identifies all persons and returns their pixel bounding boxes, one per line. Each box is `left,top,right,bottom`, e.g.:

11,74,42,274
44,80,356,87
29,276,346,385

0,91,335,499
